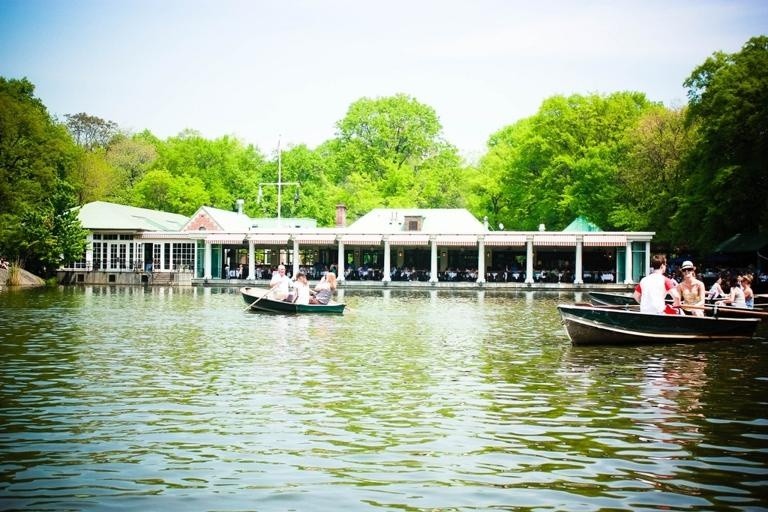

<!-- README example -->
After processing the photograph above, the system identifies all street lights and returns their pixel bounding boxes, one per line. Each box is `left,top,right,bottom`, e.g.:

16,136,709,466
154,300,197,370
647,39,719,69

256,138,303,221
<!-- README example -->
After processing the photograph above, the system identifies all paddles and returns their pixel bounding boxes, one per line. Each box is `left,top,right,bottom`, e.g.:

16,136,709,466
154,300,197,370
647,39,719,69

575,303,640,308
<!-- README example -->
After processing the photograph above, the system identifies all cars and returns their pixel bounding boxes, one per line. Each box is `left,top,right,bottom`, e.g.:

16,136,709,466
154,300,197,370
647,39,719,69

491,270,614,284
668,259,767,285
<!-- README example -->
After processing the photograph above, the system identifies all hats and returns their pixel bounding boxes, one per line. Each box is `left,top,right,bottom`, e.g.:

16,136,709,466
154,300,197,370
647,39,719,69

680,260,696,271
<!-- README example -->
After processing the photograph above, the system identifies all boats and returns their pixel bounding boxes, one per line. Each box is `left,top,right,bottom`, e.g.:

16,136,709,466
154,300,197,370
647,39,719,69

554,301,764,350
239,284,349,317
583,283,767,330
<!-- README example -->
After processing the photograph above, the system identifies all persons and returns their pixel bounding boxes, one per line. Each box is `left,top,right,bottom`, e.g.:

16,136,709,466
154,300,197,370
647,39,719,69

708,269,761,310
310,272,337,304
267,264,292,301
288,273,310,305
675,260,706,317
634,253,680,315
